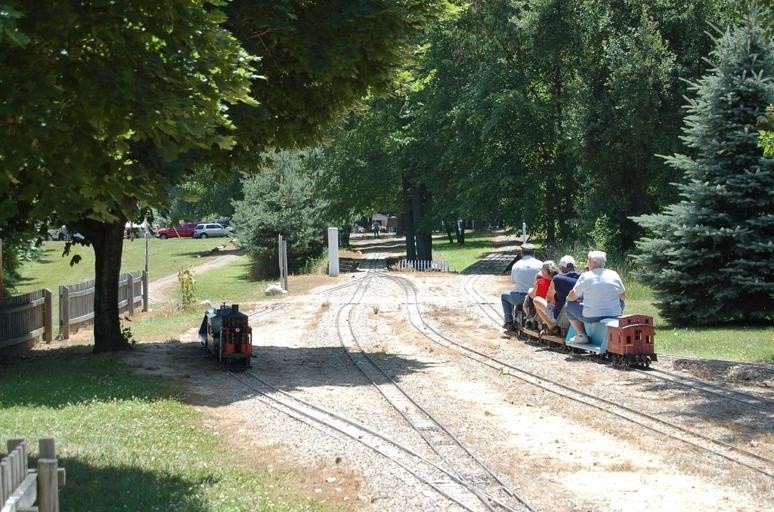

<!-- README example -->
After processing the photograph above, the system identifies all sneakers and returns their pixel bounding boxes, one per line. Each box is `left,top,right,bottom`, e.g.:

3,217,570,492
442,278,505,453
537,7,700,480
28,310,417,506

539,327,560,335
570,334,589,343
502,322,512,328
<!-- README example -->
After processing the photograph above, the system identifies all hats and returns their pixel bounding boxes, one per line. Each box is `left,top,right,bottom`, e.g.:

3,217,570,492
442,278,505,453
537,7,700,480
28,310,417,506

521,243,535,251
560,255,576,268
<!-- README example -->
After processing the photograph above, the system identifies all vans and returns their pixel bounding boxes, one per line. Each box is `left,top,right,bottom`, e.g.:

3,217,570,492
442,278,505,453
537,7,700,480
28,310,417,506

156,222,199,239
194,222,229,238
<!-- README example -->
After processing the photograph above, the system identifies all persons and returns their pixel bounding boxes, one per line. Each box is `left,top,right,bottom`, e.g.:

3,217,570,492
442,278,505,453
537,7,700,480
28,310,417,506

521,259,558,331
535,254,581,334
564,249,627,344
500,243,543,330
522,273,542,319
573,266,589,303
374,225,382,235
546,270,559,311
